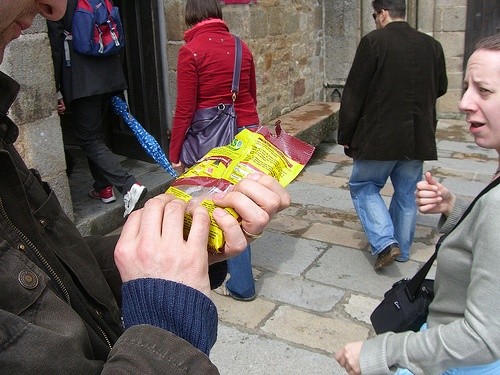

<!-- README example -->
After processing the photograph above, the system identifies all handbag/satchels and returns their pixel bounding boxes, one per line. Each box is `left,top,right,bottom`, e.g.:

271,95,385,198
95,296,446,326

370,278,435,335
180,103,238,168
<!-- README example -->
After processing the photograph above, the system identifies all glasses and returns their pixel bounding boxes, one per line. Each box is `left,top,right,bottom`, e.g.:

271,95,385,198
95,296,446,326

373,9,390,20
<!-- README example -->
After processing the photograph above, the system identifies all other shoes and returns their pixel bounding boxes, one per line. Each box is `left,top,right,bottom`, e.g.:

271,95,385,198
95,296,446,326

212,277,256,301
375,243,401,270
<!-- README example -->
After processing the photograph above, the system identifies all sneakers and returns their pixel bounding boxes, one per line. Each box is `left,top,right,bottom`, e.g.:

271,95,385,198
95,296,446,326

88,185,116,204
123,181,148,219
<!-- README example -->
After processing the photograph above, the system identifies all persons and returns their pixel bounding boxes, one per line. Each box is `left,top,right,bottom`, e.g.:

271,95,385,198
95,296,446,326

334,34,500,375
337,0,447,270
168,0,260,300
0,0,291,375
46,0,149,218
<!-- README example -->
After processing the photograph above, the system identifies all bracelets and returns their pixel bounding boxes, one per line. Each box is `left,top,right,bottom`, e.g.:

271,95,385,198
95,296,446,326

241,225,263,239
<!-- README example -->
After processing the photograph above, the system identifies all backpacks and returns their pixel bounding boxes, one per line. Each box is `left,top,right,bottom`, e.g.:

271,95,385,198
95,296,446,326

62,0,126,58
